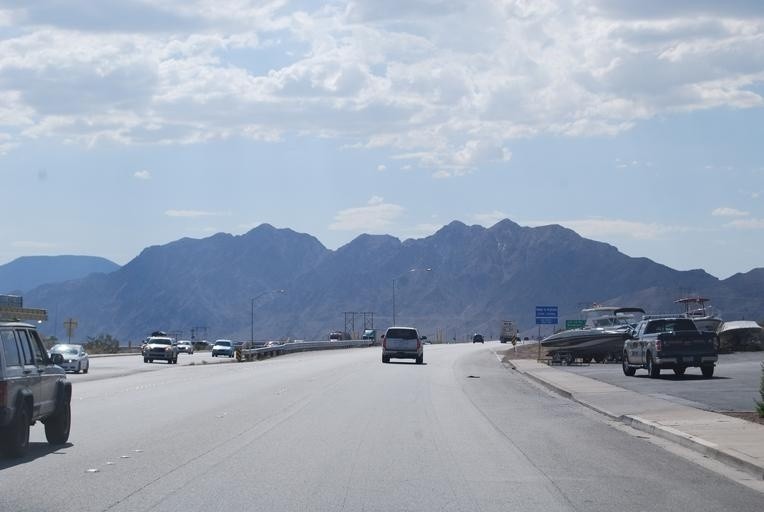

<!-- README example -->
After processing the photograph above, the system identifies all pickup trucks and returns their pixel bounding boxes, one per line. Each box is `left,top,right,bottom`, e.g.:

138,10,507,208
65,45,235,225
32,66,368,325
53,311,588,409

619,315,720,380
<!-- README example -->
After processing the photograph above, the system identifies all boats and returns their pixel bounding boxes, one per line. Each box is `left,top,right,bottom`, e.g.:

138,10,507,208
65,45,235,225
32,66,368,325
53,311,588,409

672,297,763,350
539,306,723,365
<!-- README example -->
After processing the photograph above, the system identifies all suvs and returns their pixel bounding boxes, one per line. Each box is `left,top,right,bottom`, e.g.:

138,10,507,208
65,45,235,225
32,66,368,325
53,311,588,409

471,332,485,344
380,326,427,363
0,323,74,450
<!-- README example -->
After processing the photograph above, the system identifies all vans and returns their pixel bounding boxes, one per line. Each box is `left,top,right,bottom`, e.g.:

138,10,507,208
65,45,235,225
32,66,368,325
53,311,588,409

194,341,214,350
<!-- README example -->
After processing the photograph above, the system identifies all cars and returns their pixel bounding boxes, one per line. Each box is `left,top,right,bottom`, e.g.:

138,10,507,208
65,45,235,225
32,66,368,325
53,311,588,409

211,339,235,358
139,337,193,364
48,342,89,376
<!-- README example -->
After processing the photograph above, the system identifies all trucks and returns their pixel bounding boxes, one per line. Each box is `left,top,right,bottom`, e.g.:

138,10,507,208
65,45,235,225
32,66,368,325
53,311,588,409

498,318,521,344
362,329,386,341
329,332,350,342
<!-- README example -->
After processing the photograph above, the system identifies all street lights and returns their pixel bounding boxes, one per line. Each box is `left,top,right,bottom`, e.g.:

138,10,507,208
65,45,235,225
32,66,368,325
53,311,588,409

251,289,287,348
392,265,435,327
